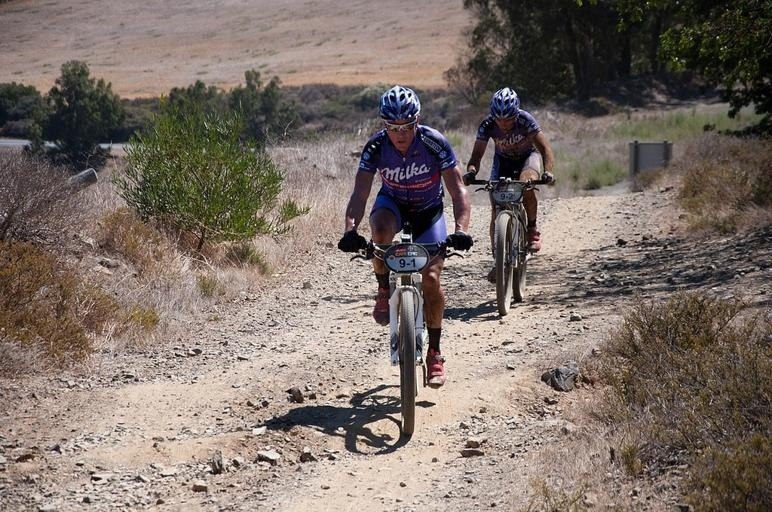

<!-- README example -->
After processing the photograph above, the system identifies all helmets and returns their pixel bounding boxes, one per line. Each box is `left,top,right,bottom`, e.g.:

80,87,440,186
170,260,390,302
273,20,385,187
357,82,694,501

376,85,421,120
490,87,520,118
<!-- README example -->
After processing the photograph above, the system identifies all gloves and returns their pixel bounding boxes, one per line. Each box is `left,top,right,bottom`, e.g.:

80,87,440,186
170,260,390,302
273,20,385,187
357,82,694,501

337,229,367,252
446,231,473,251
463,171,475,186
541,171,557,186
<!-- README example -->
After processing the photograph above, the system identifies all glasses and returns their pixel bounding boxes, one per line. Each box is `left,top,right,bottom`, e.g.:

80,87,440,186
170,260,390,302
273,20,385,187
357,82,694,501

383,116,419,131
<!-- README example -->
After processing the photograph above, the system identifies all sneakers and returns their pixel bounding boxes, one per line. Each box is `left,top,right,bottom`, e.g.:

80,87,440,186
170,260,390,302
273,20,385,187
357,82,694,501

425,349,446,389
525,230,541,251
372,286,391,326
486,268,495,285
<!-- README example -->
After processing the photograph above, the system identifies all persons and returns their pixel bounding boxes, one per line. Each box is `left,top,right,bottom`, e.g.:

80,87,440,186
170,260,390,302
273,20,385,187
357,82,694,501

341,86,471,388
462,87,554,284
